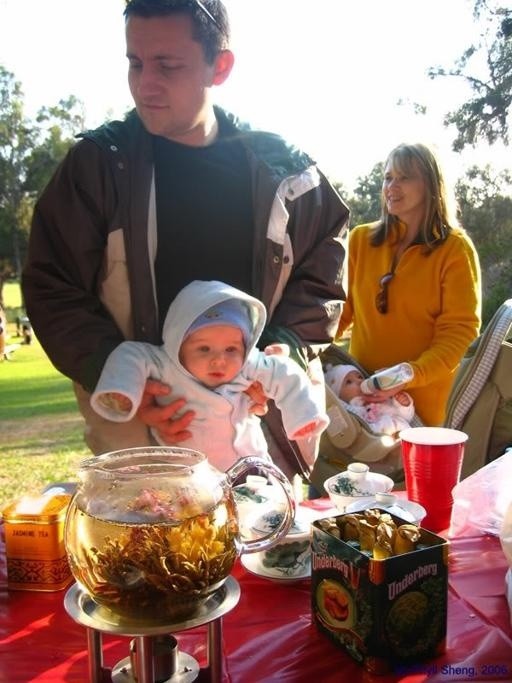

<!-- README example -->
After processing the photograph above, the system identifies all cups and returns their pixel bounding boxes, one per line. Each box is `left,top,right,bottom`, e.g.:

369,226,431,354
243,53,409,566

222,461,427,579
400,424,469,536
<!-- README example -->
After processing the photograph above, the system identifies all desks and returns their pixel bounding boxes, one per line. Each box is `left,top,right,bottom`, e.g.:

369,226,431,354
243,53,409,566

1,487,509,682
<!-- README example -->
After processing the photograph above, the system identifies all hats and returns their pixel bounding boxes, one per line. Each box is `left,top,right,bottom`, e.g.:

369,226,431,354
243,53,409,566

181,297,252,355
321,362,365,399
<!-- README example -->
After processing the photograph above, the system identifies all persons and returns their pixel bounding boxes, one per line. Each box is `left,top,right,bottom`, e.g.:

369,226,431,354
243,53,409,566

26,0,483,501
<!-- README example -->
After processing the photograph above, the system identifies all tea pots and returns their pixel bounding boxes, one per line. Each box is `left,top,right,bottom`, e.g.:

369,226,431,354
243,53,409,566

63,445,294,625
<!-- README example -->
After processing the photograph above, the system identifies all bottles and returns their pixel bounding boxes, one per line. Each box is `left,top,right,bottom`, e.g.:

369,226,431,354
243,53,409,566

360,362,414,397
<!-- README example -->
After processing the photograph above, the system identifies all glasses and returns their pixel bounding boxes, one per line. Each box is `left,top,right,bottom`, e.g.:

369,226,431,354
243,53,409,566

374,271,395,315
